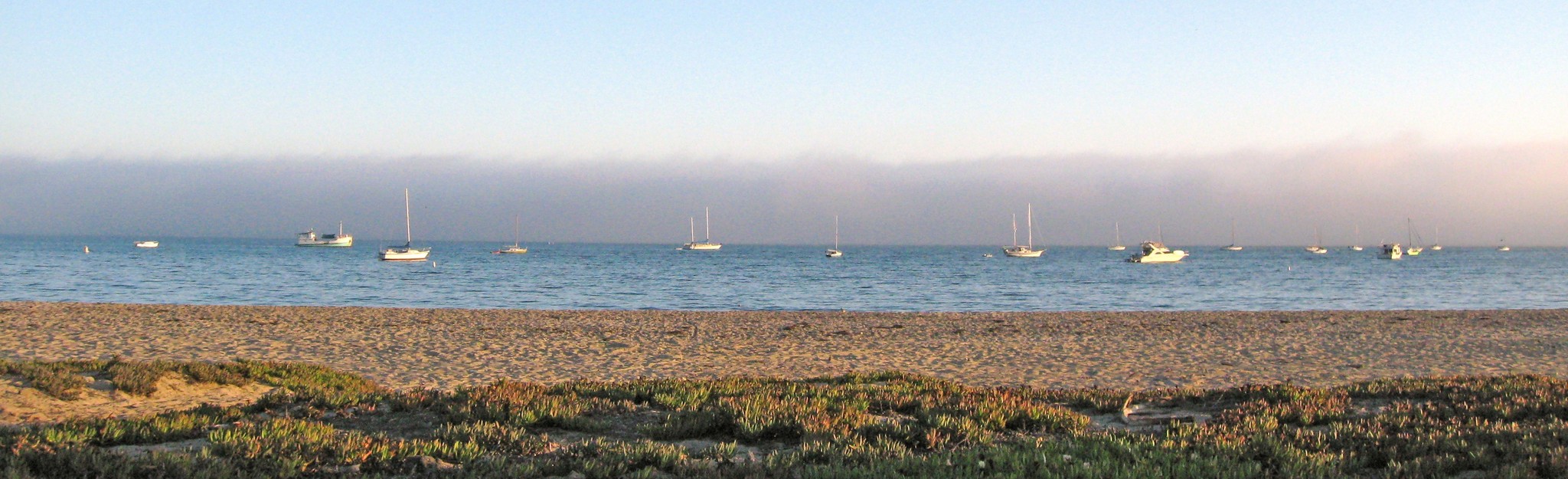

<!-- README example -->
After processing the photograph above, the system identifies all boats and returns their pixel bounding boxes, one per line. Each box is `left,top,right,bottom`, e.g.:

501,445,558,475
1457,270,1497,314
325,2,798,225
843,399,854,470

1125,241,1190,264
133,241,159,248
295,220,353,247
1304,245,1319,251
1376,242,1403,260
1312,247,1328,253
1496,239,1510,251
983,253,993,257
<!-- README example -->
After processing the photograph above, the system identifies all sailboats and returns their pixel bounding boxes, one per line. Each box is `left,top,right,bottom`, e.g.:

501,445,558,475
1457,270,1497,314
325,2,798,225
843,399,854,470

1002,202,1048,258
1347,224,1363,251
491,214,528,255
826,215,842,258
1430,228,1442,250
1406,217,1424,256
682,207,722,250
377,188,432,261
1107,222,1126,251
1220,223,1243,251
